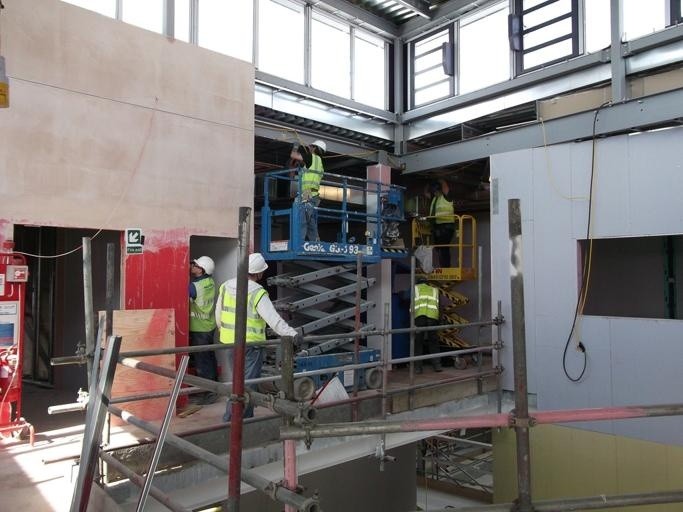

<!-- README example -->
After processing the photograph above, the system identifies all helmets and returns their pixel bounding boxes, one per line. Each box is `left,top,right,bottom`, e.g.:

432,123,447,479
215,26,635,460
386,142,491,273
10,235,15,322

193,255,215,275
308,140,327,155
247,252,268,274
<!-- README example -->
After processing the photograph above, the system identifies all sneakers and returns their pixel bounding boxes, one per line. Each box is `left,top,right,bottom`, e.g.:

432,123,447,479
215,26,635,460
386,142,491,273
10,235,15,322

176,403,203,419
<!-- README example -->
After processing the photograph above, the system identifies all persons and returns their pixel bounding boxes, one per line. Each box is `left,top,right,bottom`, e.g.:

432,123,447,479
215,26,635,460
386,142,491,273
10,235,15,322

423,178,456,267
287,139,326,256
213,251,301,422
410,277,453,374
187,254,219,406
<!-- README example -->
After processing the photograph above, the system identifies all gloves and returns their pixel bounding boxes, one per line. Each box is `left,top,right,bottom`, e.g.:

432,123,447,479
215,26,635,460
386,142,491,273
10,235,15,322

291,141,301,152
292,334,308,354
